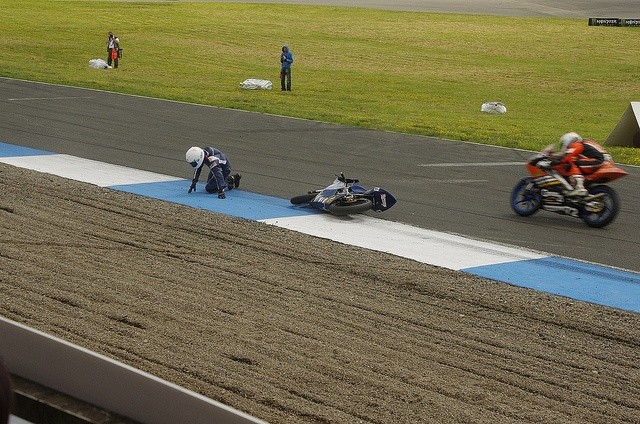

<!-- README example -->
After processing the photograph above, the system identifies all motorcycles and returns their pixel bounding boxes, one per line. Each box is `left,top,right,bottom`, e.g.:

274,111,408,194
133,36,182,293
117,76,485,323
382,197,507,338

290,171,398,215
508,143,630,229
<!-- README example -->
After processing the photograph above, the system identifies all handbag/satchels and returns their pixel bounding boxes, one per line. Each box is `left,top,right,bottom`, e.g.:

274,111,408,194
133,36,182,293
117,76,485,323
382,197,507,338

112,48,118,59
118,49,122,58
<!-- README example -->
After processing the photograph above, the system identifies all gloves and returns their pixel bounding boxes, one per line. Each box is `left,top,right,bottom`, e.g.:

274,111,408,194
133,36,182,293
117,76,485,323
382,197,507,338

218,189,226,199
187,179,198,193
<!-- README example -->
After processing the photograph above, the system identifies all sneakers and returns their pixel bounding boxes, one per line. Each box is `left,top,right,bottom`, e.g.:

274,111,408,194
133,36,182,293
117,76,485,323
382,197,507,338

227,175,235,190
565,186,588,196
234,173,241,188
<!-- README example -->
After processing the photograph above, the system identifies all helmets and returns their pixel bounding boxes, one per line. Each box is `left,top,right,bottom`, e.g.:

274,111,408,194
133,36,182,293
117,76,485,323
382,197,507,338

185,146,205,168
559,131,583,151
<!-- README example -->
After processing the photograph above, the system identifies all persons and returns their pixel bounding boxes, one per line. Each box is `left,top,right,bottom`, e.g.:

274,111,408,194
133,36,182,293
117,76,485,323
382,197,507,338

537,131,615,200
106,31,120,69
184,146,243,199
279,43,293,92
